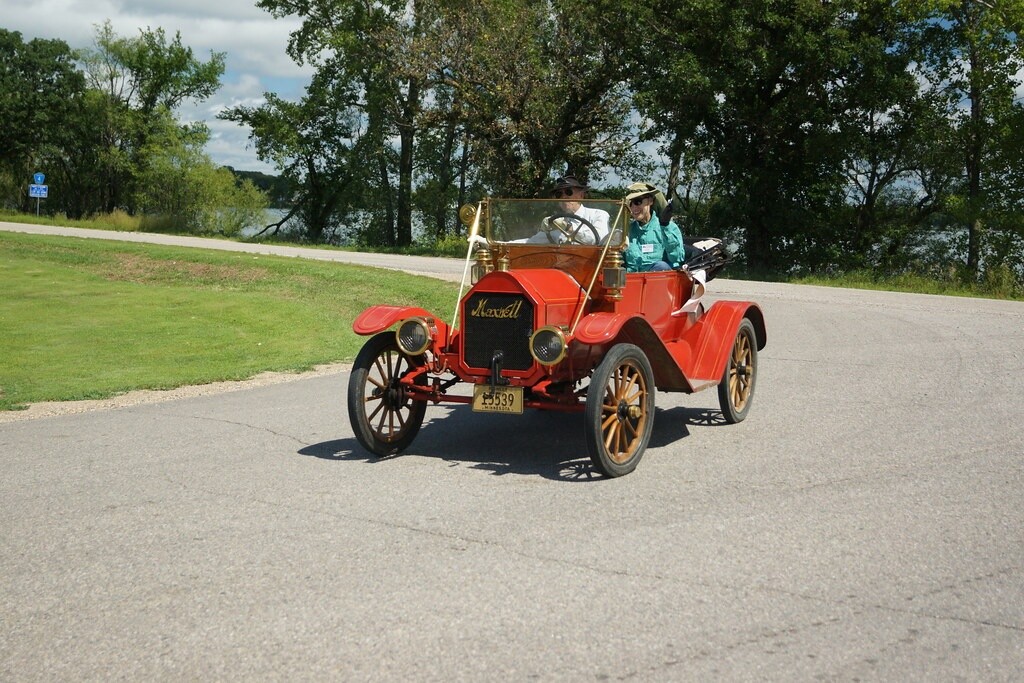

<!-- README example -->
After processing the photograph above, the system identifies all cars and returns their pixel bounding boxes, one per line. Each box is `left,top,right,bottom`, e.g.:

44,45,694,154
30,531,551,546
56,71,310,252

346,198,766,480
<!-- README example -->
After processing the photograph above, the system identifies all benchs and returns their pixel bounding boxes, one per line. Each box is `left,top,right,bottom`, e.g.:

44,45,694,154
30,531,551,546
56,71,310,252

662,243,709,277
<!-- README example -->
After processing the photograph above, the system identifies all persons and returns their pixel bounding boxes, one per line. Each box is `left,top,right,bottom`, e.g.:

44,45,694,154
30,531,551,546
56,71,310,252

466,174,610,251
598,181,687,272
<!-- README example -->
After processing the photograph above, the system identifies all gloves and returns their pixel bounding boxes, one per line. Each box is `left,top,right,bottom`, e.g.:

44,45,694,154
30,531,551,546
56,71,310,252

467,234,487,251
541,216,567,234
671,266,682,272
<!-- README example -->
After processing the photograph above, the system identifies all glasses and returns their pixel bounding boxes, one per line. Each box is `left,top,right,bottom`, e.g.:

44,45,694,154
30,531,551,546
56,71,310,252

630,196,649,207
555,189,579,198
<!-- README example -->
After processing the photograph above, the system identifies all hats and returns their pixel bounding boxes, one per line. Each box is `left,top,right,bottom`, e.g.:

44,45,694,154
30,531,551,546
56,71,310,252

548,176,579,195
624,182,660,200
